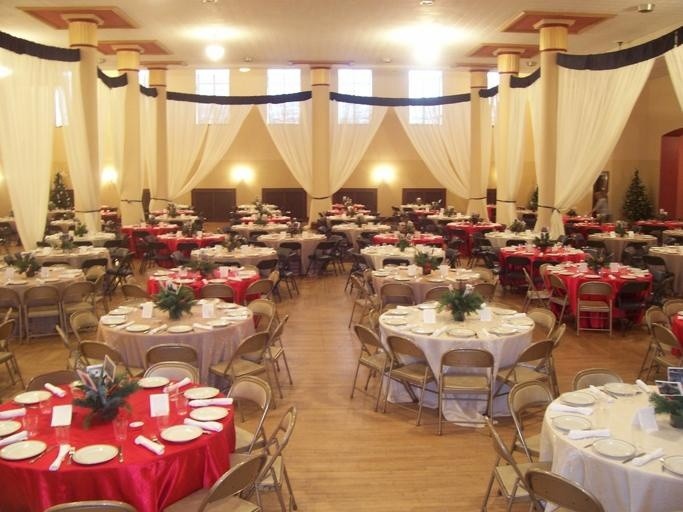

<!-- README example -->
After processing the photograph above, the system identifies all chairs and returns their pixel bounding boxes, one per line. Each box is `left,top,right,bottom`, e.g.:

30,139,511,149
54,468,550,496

484,215,682,336
1,223,299,511
483,370,682,511
636,299,682,384
256,201,506,293
343,263,568,437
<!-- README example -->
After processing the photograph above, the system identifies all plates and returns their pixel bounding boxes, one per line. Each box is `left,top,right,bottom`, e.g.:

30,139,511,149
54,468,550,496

552,381,682,476
498,197,681,238
0,377,230,466
0,201,318,339
502,240,648,279
328,203,497,282
387,299,533,335
650,239,682,254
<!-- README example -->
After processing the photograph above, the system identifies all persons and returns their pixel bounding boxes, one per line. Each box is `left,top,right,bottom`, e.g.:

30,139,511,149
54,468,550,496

588,189,610,223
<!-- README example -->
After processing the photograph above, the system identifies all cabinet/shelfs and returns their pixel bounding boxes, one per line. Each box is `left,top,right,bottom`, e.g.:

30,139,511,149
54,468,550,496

257,186,309,223
399,186,448,210
189,187,238,224
329,187,378,216
